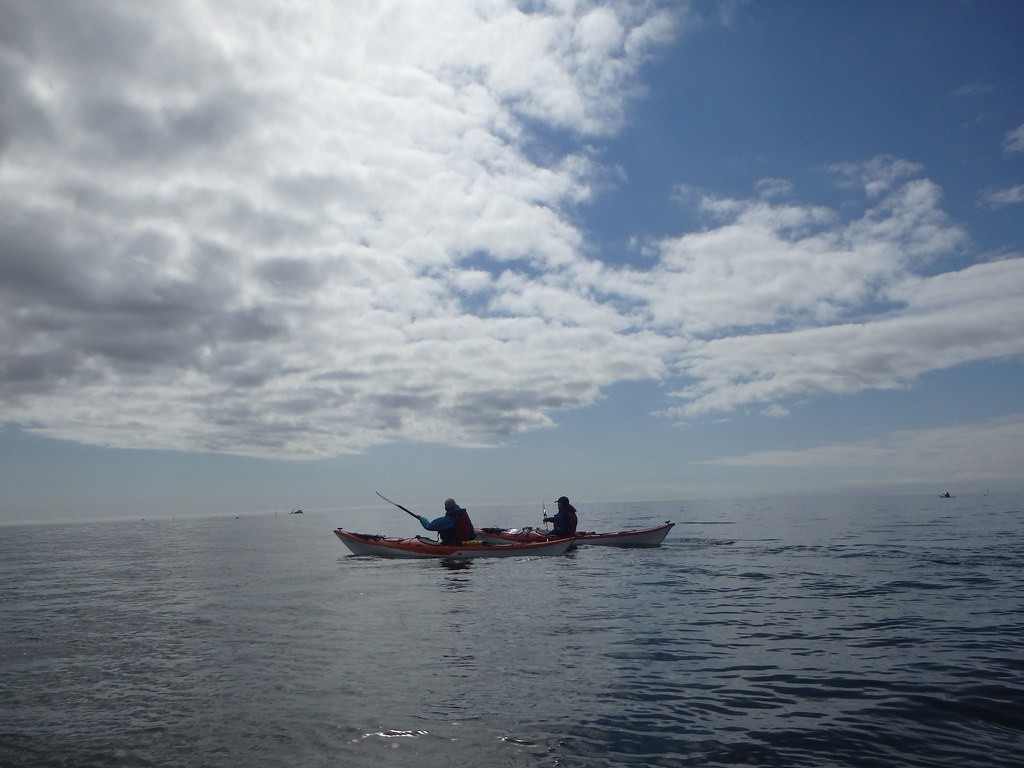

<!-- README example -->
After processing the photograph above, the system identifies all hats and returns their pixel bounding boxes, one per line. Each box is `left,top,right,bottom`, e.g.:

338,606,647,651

445,499,455,509
554,496,569,504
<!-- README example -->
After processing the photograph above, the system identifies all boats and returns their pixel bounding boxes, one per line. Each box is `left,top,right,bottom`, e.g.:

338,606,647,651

333,527,575,557
472,520,677,546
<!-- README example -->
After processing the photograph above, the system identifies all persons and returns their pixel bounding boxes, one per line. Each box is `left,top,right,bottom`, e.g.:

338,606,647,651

416,498,476,546
542,496,577,537
945,492,949,496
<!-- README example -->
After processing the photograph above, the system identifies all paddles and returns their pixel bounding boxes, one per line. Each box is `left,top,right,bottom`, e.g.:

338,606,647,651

375,490,420,521
541,500,550,542
